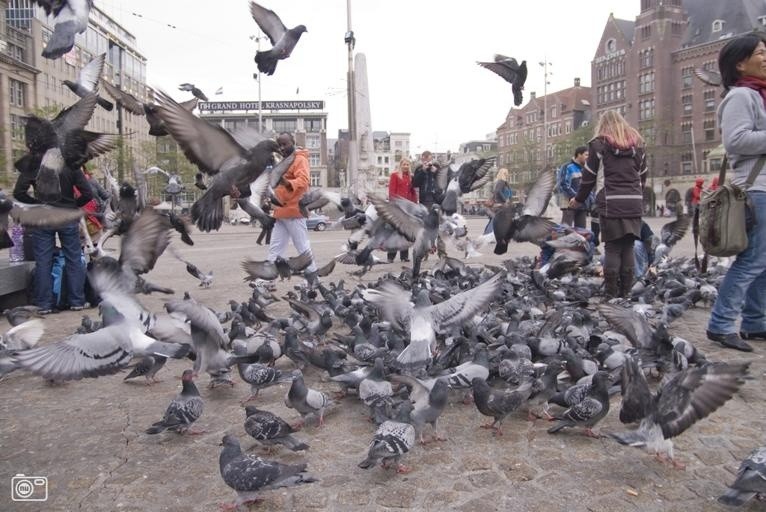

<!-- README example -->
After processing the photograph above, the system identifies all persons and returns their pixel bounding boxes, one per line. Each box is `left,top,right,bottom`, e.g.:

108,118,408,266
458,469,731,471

0,161,136,312
556,145,595,231
589,191,601,248
266,130,315,273
493,166,513,206
567,108,649,302
410,150,441,204
639,174,725,219
255,183,274,247
706,33,766,354
384,156,417,265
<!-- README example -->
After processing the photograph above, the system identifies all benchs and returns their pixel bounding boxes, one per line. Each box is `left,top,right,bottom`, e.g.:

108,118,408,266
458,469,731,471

1,226,37,298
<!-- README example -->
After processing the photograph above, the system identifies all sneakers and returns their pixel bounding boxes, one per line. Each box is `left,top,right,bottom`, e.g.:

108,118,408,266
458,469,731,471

69,301,93,311
34,305,62,316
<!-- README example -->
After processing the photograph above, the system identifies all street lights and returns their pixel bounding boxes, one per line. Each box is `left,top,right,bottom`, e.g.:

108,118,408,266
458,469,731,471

247,34,271,138
538,60,552,168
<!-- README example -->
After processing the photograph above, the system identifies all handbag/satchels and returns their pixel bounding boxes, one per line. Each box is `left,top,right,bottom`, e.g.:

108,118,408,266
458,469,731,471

26,246,86,309
688,183,752,257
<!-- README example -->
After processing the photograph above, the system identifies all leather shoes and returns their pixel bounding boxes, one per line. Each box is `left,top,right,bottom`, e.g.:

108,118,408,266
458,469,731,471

706,327,753,355
738,328,766,341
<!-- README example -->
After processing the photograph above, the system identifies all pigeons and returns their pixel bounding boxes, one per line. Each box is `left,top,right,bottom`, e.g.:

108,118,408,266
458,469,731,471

2,305,43,324
199,269,214,288
33,0,90,58
179,83,209,102
249,0,309,76
59,53,119,110
1,319,45,351
1,358,18,378
217,158,766,510
165,294,235,392
14,92,128,203
124,354,169,382
116,204,175,296
20,264,190,382
118,181,137,234
147,166,186,195
477,52,529,107
148,87,283,233
188,262,202,280
81,312,102,331
146,370,203,437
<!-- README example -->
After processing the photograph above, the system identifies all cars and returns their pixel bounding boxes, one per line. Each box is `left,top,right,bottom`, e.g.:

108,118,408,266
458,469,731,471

304,211,331,232
230,216,250,227
